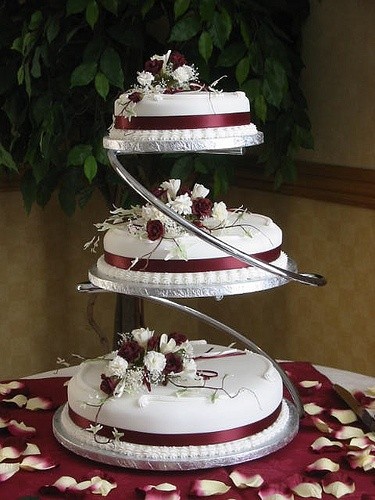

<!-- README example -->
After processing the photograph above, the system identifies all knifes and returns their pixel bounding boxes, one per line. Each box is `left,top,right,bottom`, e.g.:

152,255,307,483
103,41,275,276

331,381,375,434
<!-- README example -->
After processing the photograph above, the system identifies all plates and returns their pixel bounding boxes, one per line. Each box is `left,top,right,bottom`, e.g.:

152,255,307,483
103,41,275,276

102,129,265,154
50,397,301,473
87,255,299,299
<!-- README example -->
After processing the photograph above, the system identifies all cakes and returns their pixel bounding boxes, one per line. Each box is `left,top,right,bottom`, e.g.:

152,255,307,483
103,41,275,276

106,50,258,141
60,327,290,459
82,177,289,286
